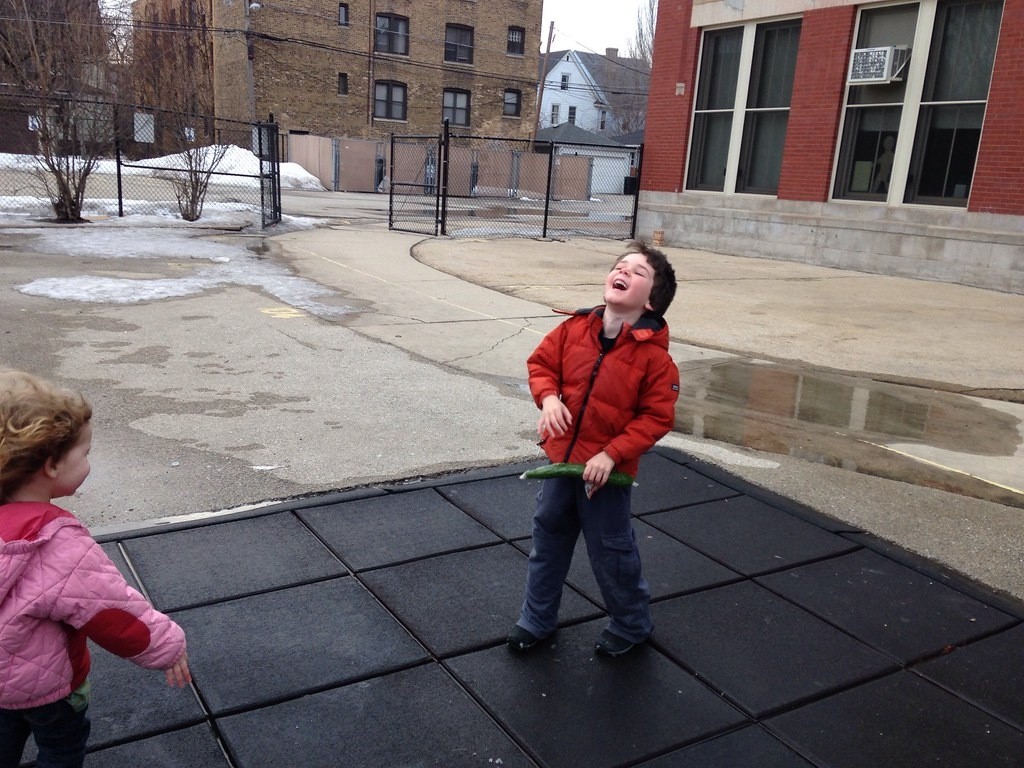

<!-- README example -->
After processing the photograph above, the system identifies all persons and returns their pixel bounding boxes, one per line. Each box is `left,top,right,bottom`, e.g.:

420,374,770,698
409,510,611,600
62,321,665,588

506,236,682,660
0,370,192,768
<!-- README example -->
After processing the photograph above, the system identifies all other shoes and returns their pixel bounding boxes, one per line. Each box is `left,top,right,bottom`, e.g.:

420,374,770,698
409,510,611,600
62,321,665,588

506,625,537,649
594,624,657,658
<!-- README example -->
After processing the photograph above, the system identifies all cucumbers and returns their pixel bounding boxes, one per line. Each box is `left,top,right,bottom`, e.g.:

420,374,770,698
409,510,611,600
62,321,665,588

523,462,633,488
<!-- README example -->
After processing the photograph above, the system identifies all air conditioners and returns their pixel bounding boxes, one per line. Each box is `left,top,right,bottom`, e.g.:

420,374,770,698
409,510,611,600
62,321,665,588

849,46,906,82
